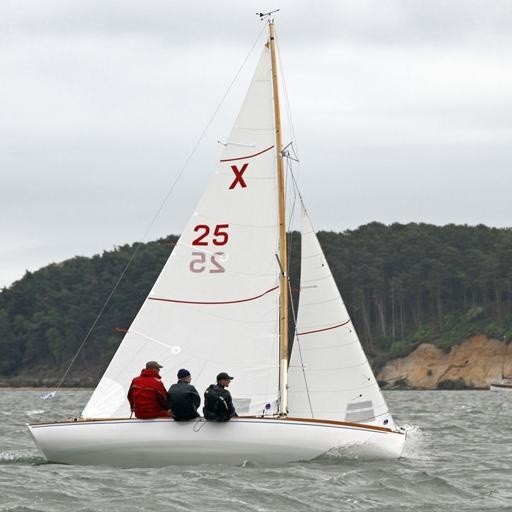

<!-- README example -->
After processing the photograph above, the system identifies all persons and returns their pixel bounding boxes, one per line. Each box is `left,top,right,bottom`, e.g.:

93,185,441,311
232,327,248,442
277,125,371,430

204,373,238,422
166,369,200,421
127,361,172,419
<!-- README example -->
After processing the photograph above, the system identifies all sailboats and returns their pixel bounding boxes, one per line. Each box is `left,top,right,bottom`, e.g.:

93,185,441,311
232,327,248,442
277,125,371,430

23,7,409,465
489,335,511,391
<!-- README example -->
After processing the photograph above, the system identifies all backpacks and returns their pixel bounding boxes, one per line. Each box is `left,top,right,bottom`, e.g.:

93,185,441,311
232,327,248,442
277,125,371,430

205,388,235,424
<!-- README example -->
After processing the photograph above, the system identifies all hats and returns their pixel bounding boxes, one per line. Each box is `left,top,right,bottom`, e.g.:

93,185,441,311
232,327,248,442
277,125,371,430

145,360,164,370
177,368,190,379
215,372,235,381
